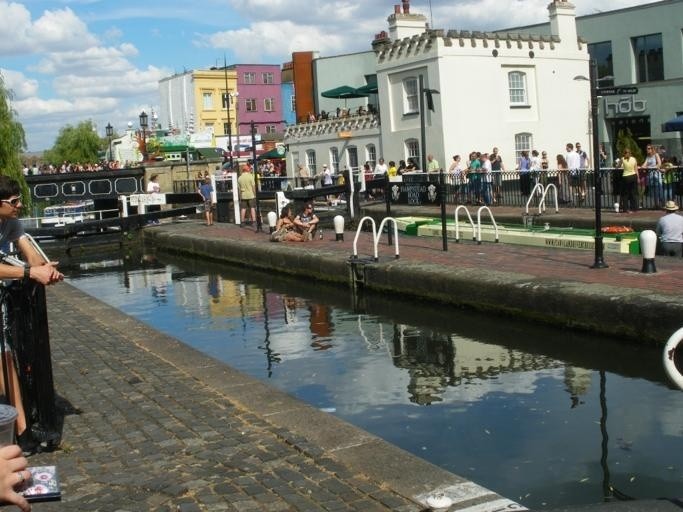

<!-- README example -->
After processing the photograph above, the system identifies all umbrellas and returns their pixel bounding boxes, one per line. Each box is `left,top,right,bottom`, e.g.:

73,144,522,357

357,82,378,102
321,85,368,108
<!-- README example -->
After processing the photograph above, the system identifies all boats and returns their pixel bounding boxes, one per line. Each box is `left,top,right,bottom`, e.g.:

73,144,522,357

39,199,96,228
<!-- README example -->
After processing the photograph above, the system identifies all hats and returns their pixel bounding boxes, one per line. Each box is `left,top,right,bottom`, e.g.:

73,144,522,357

662,201,679,211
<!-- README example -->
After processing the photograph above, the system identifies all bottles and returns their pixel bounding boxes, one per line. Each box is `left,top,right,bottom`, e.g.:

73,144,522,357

319,227,323,239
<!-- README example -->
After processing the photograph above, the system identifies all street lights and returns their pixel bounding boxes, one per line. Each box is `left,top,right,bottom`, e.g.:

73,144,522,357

105,122,114,161
137,111,148,159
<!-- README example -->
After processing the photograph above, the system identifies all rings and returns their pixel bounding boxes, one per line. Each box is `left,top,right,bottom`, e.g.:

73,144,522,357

17,471,25,483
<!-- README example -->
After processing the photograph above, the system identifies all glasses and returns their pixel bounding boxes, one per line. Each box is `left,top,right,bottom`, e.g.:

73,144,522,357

0,192,25,207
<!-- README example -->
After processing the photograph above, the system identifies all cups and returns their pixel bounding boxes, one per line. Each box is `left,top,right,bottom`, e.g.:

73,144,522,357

613,203,619,213
543,222,551,231
616,234,624,242
0,404,17,450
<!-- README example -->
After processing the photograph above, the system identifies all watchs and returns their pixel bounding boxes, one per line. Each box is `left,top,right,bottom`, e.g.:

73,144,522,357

21,263,31,280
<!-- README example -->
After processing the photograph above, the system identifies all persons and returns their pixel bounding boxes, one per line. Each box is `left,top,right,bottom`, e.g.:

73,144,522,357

22,160,119,176
448,147,504,205
0,444,32,511
147,173,160,225
557,142,589,201
656,201,682,255
612,144,682,213
271,202,320,242
196,159,284,226
307,158,420,202
426,155,439,181
0,173,64,451
516,150,548,196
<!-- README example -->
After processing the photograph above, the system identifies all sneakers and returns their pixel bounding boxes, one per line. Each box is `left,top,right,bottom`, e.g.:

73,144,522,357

16,426,60,451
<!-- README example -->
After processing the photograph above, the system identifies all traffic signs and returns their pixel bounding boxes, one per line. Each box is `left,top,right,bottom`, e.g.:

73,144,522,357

252,139,264,144
594,87,638,95
244,146,253,152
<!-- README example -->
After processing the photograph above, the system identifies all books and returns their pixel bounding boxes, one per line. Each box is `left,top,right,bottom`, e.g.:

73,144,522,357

9,465,60,499
